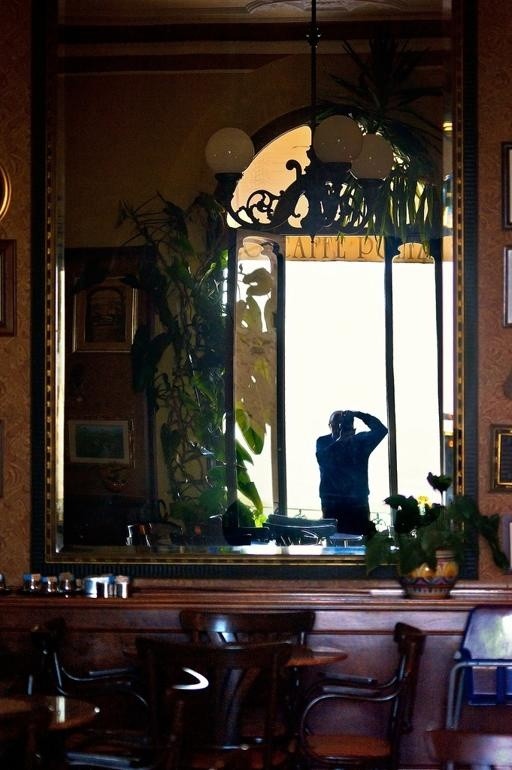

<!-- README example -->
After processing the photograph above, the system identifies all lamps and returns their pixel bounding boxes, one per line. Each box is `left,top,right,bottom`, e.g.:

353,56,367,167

206,1,393,242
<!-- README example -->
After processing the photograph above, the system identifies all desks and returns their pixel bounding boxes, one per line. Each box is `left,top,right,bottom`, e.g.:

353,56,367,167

182,608,318,643
291,646,344,674
45,696,95,730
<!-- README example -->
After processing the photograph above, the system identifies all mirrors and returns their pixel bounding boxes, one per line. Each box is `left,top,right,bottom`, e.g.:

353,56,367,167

30,34,481,580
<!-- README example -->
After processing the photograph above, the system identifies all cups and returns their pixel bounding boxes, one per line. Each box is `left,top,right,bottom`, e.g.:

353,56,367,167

23,574,129,599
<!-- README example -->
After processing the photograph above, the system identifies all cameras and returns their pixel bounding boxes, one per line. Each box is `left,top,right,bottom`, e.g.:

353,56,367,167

338,416,355,435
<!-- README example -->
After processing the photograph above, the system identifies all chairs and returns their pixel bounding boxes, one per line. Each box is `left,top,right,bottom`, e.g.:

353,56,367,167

293,621,425,765
262,512,363,546
43,615,144,725
432,603,512,769
138,634,290,765
0,697,50,768
66,667,209,768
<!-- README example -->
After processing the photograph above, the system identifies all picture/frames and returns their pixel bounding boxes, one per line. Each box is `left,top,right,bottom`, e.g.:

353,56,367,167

63,413,137,465
0,238,17,338
487,422,511,493
502,244,512,326
72,275,137,355
500,141,512,231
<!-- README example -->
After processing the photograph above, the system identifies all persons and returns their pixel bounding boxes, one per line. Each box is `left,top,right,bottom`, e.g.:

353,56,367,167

315,410,389,534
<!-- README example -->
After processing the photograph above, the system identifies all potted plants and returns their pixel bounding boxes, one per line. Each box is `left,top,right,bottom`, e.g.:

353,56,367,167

367,472,509,599
